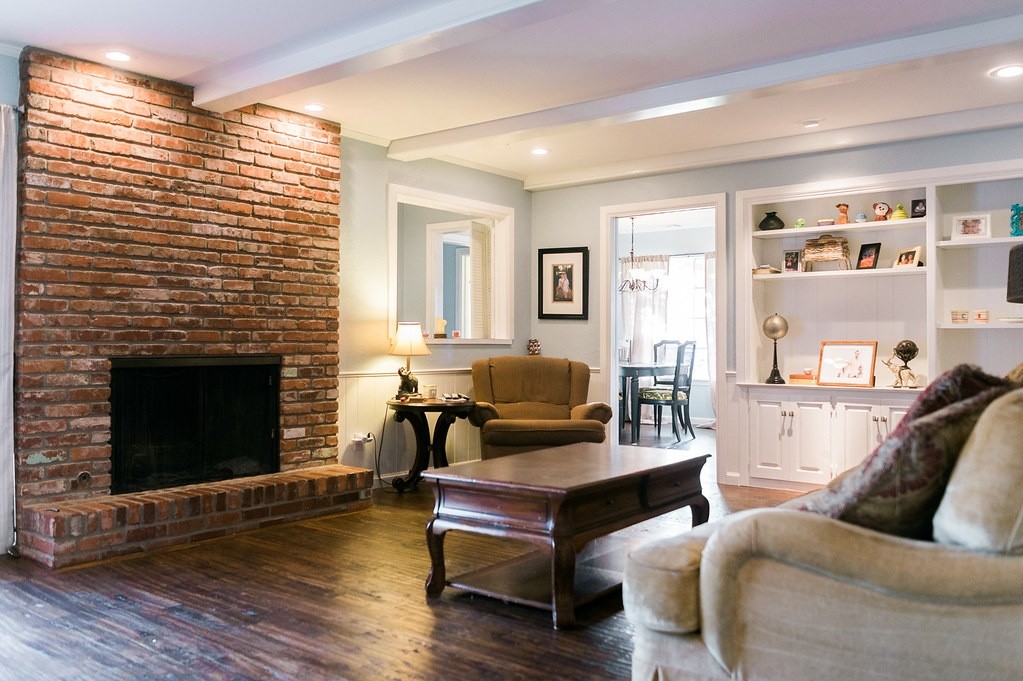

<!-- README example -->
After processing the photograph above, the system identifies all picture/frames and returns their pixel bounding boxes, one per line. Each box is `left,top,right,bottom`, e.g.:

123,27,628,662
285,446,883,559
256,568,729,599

893,246,923,269
817,340,877,388
951,214,992,241
856,243,881,269
782,250,802,272
538,246,589,320
911,199,926,219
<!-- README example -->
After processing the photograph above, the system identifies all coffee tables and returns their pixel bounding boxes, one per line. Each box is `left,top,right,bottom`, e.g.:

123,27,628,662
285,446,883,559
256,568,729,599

421,442,712,632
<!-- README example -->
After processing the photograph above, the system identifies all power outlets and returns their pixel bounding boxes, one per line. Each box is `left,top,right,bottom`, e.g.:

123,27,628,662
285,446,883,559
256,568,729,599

353,434,364,451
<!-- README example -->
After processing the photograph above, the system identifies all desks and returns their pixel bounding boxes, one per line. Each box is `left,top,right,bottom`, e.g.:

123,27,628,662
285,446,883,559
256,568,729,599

386,399,476,491
618,363,690,445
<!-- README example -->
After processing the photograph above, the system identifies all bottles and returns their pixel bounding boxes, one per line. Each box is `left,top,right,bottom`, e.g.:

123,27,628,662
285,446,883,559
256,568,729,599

759,212,785,230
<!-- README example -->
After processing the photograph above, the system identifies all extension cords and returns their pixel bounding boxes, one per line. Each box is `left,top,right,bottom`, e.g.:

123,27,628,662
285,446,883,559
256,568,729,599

355,433,373,442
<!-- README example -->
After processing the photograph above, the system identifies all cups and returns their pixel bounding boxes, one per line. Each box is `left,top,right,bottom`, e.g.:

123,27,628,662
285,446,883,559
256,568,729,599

423,385,437,398
974,310,989,323
952,311,969,323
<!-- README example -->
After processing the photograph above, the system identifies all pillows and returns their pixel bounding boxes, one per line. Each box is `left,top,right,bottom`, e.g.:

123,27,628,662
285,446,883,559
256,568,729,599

800,364,1019,506
787,386,1007,533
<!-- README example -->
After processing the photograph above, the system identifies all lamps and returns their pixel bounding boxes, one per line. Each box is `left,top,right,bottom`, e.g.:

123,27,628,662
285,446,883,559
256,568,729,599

617,216,660,294
388,322,432,402
1007,242,1023,305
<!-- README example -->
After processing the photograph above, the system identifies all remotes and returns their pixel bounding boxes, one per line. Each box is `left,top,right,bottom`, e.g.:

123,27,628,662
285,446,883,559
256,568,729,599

458,394,470,400
452,394,459,399
442,393,451,399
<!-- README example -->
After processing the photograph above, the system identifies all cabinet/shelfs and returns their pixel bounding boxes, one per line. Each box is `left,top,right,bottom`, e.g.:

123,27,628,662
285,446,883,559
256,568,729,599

726,159,1023,494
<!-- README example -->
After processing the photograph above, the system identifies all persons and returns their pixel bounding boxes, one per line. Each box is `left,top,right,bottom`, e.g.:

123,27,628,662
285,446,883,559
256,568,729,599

557,273,569,298
861,249,874,267
915,202,925,212
959,220,982,234
838,351,863,378
899,254,913,264
786,256,792,266
888,340,919,387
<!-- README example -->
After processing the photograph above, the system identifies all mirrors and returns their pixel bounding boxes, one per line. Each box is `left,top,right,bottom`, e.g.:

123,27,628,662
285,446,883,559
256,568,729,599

387,183,515,345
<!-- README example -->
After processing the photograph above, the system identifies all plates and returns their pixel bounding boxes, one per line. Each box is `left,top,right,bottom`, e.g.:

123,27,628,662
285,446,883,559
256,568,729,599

998,318,1023,322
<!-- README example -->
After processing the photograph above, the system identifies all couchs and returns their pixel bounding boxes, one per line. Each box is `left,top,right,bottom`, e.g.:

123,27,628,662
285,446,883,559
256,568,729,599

468,356,613,460
619,358,1023,681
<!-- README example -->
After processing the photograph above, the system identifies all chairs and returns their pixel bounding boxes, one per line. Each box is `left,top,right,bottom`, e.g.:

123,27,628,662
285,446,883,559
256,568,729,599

618,339,696,442
